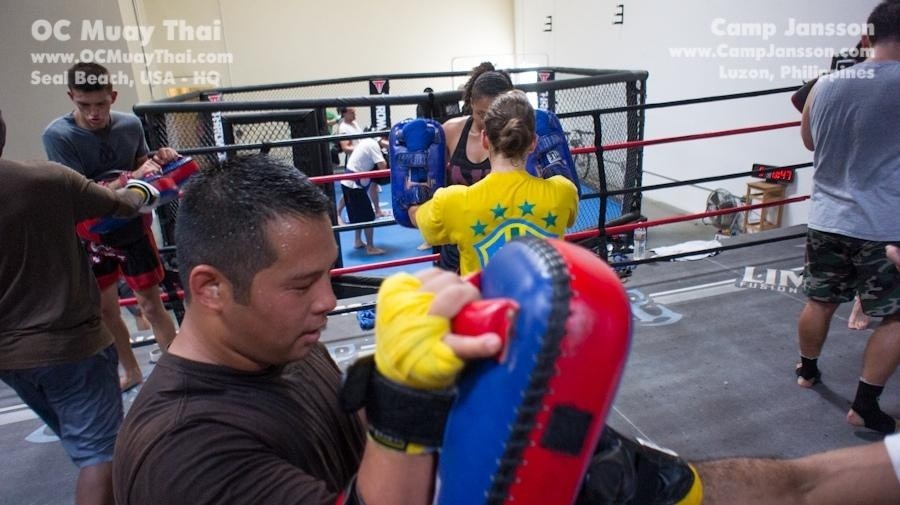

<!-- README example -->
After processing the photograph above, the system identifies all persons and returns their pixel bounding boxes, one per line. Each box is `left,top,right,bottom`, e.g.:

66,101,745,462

0,0,900,504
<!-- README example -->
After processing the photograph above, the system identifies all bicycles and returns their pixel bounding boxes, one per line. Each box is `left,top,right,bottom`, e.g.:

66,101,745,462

559,127,596,181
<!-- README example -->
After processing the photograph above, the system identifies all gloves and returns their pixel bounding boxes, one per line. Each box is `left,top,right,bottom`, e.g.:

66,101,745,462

534,109,561,160
397,118,436,168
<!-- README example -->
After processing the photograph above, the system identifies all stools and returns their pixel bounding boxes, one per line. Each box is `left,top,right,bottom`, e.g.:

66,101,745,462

743,182,785,232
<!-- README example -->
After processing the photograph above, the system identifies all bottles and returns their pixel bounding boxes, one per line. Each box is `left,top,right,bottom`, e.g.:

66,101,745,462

633,222,645,260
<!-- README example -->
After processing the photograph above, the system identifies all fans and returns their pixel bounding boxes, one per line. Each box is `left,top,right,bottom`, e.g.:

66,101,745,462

703,188,741,237
